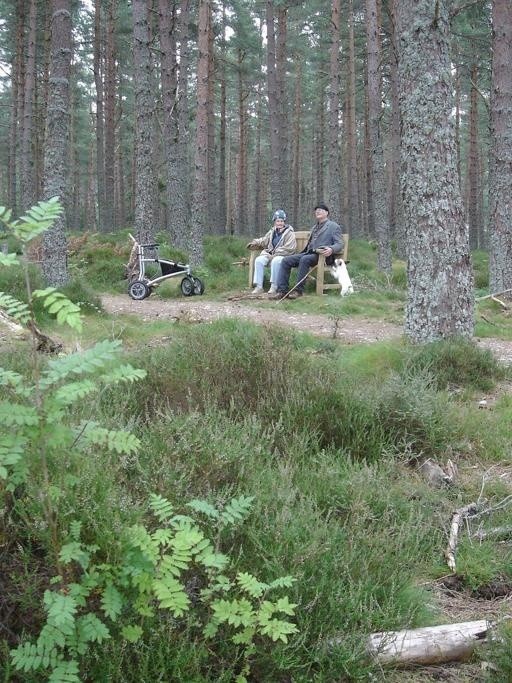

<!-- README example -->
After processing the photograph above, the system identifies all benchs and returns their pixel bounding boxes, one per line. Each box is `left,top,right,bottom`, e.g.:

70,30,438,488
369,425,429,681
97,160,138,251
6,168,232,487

248,231,349,297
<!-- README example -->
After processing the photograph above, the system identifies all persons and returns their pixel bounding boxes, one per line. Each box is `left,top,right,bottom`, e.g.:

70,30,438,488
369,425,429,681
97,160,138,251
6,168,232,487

266,203,346,299
244,209,298,297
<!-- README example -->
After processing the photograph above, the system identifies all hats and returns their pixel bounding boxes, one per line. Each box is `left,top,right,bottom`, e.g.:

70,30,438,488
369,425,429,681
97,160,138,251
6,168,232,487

271,210,288,221
315,205,329,216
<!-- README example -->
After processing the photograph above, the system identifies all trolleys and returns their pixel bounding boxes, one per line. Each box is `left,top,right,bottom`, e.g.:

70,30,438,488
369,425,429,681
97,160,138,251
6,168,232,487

120,231,210,301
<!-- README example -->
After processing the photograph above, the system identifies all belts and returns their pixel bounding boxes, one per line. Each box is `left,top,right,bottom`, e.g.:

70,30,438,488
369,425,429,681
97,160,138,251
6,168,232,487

309,250,317,254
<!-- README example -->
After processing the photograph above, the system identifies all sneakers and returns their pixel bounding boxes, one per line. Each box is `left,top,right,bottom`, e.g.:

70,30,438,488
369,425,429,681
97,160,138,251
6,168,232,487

268,287,276,294
273,292,289,300
288,289,303,299
251,287,264,294
